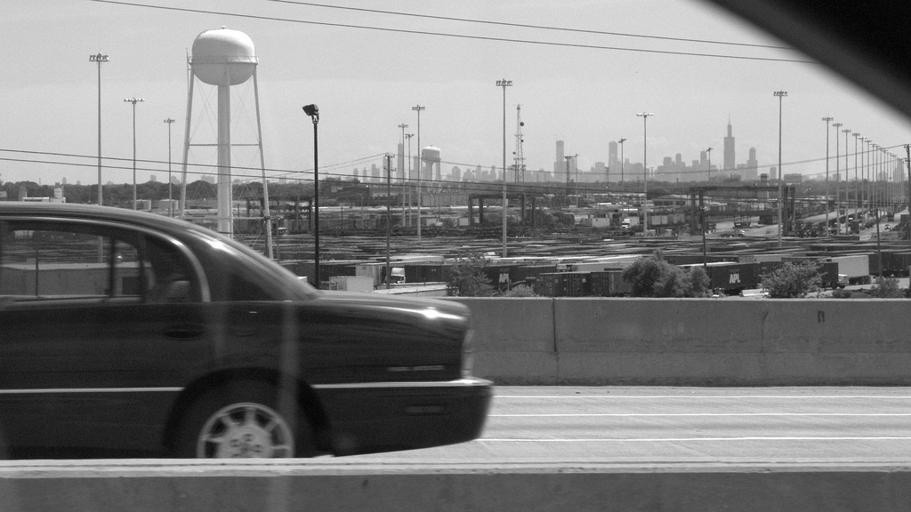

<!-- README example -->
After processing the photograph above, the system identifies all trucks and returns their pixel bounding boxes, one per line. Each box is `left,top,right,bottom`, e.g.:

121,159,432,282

384,267,406,283
819,255,870,289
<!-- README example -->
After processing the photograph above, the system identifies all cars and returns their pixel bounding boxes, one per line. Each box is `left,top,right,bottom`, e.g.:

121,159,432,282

0,201,492,460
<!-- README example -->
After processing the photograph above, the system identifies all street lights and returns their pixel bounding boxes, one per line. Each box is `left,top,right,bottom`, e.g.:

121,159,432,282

162,117,176,217
565,154,579,197
495,76,513,257
302,103,320,289
124,95,144,211
88,53,110,262
637,112,655,240
706,147,713,181
618,137,627,185
396,105,426,242
822,113,904,240
773,89,788,247
